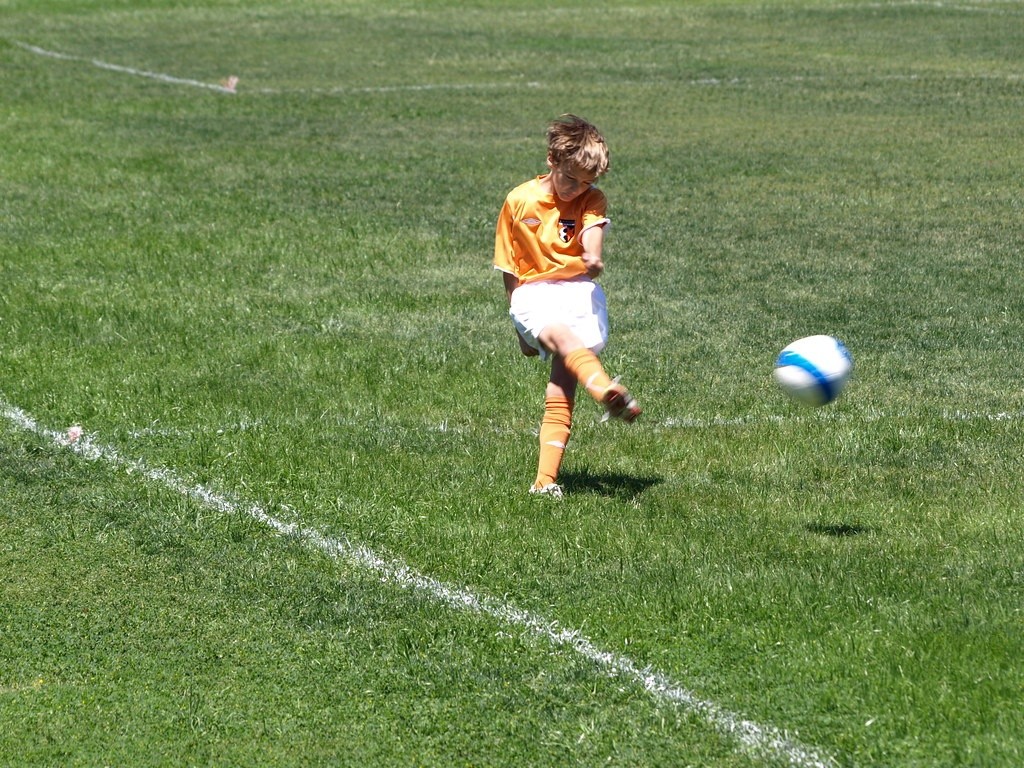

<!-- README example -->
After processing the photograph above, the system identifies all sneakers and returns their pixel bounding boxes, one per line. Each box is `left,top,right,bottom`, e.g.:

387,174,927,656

602,375,642,425
528,483,563,499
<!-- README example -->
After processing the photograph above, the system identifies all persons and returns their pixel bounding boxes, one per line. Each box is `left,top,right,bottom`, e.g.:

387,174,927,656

493,113,640,500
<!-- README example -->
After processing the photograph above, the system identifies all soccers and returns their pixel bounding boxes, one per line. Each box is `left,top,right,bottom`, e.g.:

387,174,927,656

774,334,856,405
